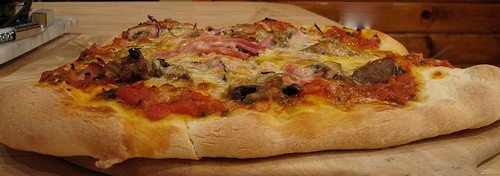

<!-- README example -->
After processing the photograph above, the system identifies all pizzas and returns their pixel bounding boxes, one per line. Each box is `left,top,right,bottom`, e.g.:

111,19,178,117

0,14,500,171
